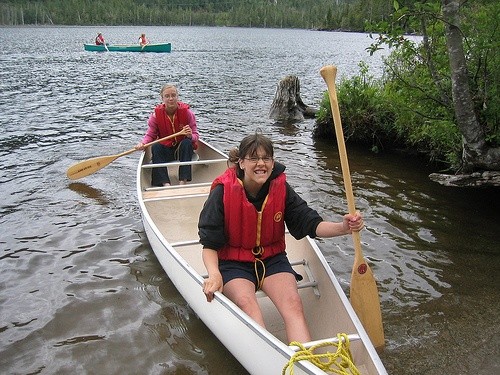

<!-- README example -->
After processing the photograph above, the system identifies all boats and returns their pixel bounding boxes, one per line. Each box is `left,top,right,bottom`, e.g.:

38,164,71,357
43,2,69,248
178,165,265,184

137,136,389,375
83,43,172,53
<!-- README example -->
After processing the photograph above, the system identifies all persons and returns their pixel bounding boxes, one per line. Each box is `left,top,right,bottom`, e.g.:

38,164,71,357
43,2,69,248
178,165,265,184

134,85,198,187
95,32,109,45
137,33,150,50
198,135,363,360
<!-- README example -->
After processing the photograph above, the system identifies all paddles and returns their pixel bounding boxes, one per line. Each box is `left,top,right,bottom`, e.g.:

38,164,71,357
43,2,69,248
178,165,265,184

320,67,386,351
66,128,186,180
104,43,110,52
142,42,150,50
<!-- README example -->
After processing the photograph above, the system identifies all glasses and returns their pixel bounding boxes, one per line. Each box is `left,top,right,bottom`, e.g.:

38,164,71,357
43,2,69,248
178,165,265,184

240,154,273,164
